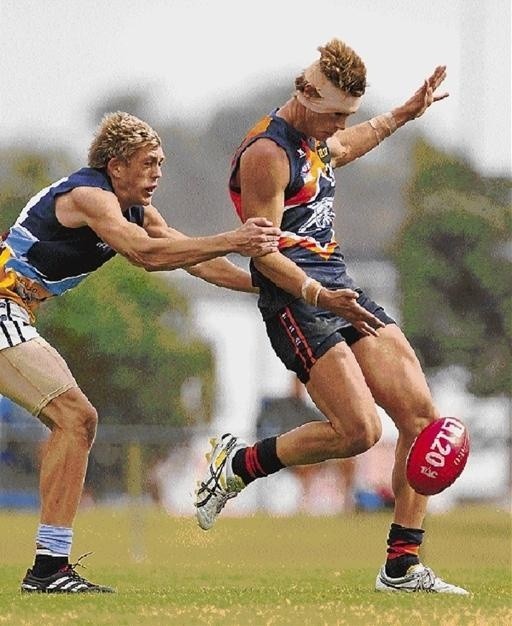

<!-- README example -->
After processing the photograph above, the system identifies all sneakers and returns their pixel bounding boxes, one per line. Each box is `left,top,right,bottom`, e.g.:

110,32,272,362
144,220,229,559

20,551,115,593
375,560,471,596
194,432,250,530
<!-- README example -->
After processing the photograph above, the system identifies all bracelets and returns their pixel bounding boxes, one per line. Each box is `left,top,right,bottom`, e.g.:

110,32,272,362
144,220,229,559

368,112,398,147
300,277,325,308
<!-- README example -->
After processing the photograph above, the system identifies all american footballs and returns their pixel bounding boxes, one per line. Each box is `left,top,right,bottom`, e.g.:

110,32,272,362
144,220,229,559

405,417,470,496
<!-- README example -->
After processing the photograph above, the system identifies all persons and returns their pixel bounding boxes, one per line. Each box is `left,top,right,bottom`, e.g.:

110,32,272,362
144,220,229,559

1,108,283,595
193,37,475,597
